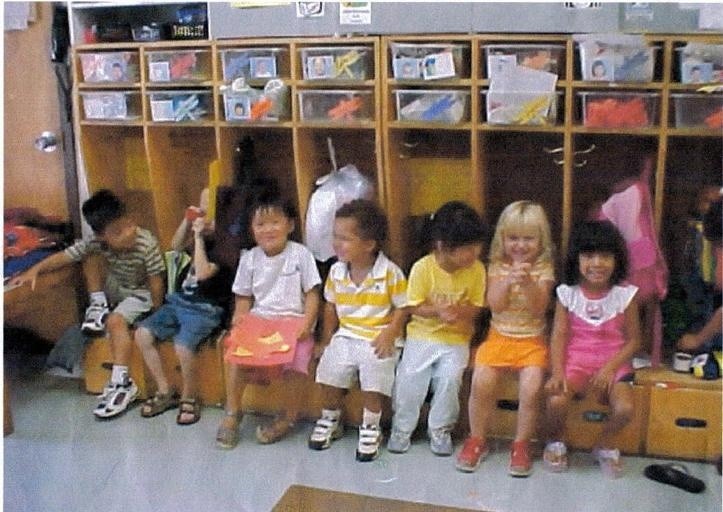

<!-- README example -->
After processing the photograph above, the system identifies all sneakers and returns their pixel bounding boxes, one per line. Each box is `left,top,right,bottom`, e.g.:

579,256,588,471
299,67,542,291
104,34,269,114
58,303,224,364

454,434,490,473
592,443,623,480
509,437,535,479
387,418,414,453
542,441,569,472
92,376,143,419
426,420,454,456
308,408,346,450
79,300,109,337
355,423,383,462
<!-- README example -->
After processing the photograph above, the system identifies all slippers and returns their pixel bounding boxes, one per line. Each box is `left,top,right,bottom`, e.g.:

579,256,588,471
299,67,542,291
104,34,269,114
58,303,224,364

644,461,706,494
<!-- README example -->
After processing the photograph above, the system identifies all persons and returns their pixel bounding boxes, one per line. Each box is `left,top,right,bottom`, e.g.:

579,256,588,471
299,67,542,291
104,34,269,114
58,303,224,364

591,62,608,81
455,199,557,476
308,199,409,462
135,184,239,425
402,63,414,76
235,103,246,118
660,195,722,354
254,62,270,79
387,200,486,455
544,221,642,479
689,68,703,83
312,57,327,78
8,190,167,418
215,192,323,450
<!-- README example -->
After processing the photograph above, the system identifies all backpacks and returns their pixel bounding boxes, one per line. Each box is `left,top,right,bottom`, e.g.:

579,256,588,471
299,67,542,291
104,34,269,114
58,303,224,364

588,156,673,308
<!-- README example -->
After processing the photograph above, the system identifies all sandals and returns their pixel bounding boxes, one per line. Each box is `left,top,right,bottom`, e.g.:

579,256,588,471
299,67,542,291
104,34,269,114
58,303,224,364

176,392,203,425
217,404,243,448
141,387,180,418
254,408,304,443
